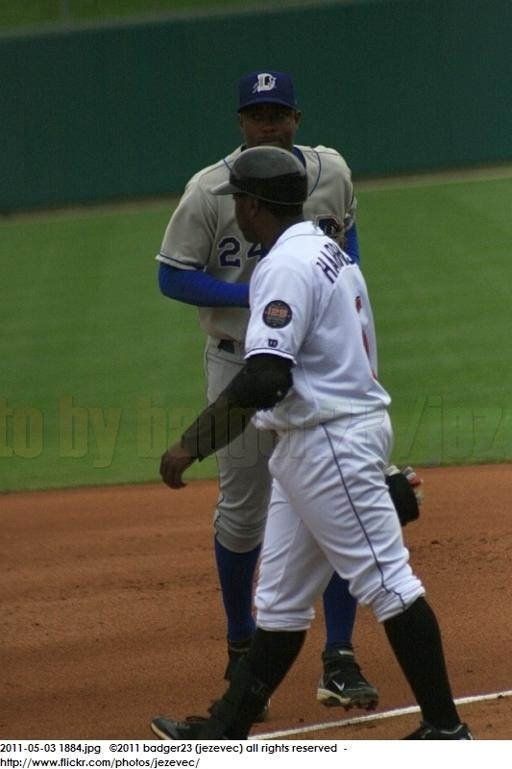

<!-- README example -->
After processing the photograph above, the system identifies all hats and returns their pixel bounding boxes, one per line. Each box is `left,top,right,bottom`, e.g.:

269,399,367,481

237,70,297,112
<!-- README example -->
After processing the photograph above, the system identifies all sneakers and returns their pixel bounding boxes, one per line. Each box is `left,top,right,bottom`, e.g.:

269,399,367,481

150,719,247,741
401,722,471,740
317,650,378,710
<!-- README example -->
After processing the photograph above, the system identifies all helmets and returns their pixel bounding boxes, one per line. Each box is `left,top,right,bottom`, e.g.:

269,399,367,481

210,144,308,204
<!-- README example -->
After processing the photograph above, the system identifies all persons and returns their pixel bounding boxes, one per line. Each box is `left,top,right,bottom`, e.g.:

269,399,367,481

155,66,379,720
150,144,480,740
